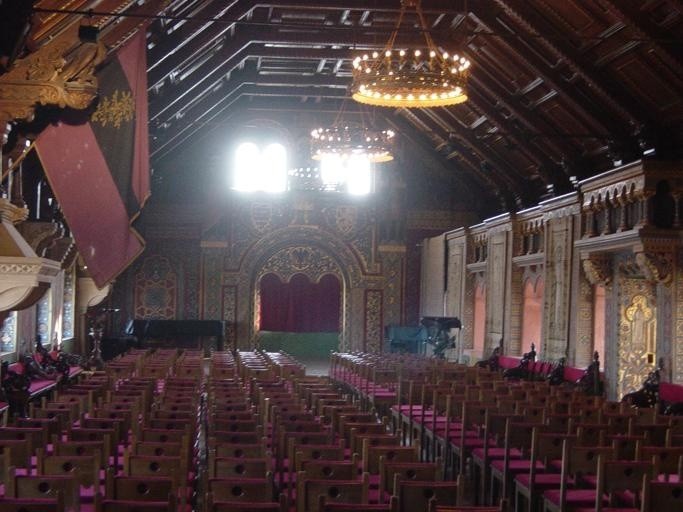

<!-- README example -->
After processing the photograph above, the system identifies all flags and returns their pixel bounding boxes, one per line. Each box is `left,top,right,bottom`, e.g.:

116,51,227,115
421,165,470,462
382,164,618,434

35,29,150,291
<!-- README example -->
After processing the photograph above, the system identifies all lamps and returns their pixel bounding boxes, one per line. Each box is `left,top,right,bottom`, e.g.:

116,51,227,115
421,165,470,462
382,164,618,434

348,1,472,110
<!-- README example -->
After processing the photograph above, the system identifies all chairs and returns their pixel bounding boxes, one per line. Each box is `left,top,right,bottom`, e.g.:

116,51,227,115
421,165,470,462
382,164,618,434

0,347,683,512
